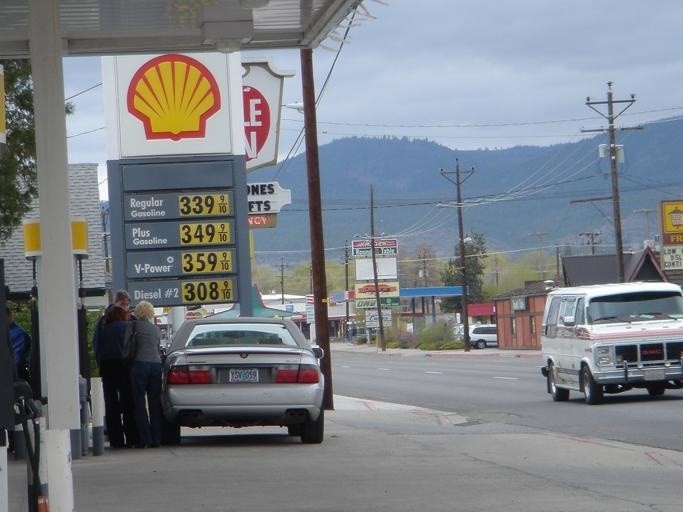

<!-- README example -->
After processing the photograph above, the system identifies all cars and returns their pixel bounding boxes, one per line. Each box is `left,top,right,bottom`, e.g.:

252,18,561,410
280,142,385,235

161,317,325,445
454,324,498,348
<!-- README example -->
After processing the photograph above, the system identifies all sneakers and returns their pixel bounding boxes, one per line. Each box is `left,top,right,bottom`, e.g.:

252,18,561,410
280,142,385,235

110,436,165,449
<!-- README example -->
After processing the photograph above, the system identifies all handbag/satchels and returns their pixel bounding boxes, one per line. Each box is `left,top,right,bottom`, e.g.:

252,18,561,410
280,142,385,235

122,320,136,369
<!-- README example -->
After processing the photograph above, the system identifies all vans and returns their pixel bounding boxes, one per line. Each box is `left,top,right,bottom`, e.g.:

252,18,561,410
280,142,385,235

541,282,683,405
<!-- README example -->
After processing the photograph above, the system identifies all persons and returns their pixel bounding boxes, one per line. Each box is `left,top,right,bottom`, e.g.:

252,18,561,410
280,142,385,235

92,289,165,448
5,305,32,377
